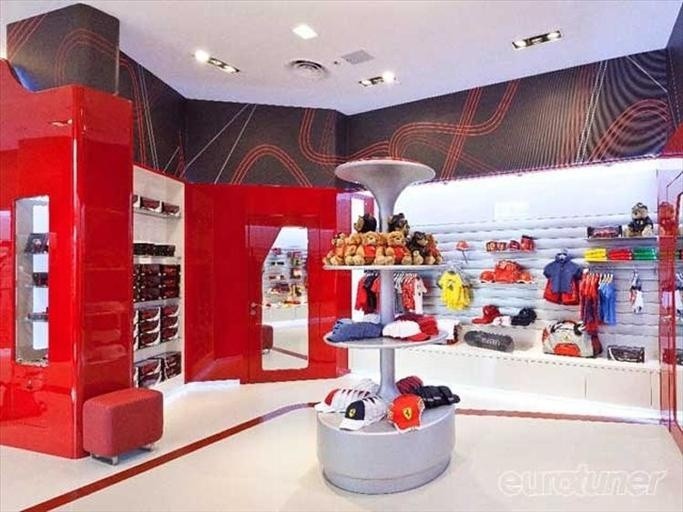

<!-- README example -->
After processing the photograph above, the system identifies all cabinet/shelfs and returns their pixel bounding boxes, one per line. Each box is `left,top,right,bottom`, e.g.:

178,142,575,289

132,205,181,390
312,158,459,496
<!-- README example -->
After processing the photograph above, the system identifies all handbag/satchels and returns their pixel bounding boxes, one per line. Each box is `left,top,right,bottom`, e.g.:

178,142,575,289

542,318,603,358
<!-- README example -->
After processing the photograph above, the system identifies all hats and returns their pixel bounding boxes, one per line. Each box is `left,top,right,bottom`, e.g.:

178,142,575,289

480,272,494,283
388,394,424,432
327,319,380,342
382,320,431,342
517,273,530,283
315,385,372,414
396,375,424,394
417,386,459,409
340,395,386,432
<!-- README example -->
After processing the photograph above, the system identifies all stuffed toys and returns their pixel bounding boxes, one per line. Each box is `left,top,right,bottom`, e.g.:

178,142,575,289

320,208,445,266
656,200,679,237
625,200,656,237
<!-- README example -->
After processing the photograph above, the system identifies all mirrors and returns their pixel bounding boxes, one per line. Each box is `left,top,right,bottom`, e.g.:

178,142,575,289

671,192,682,434
259,227,310,374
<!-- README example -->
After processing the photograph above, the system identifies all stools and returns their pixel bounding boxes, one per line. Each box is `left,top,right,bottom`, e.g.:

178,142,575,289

80,386,164,467
